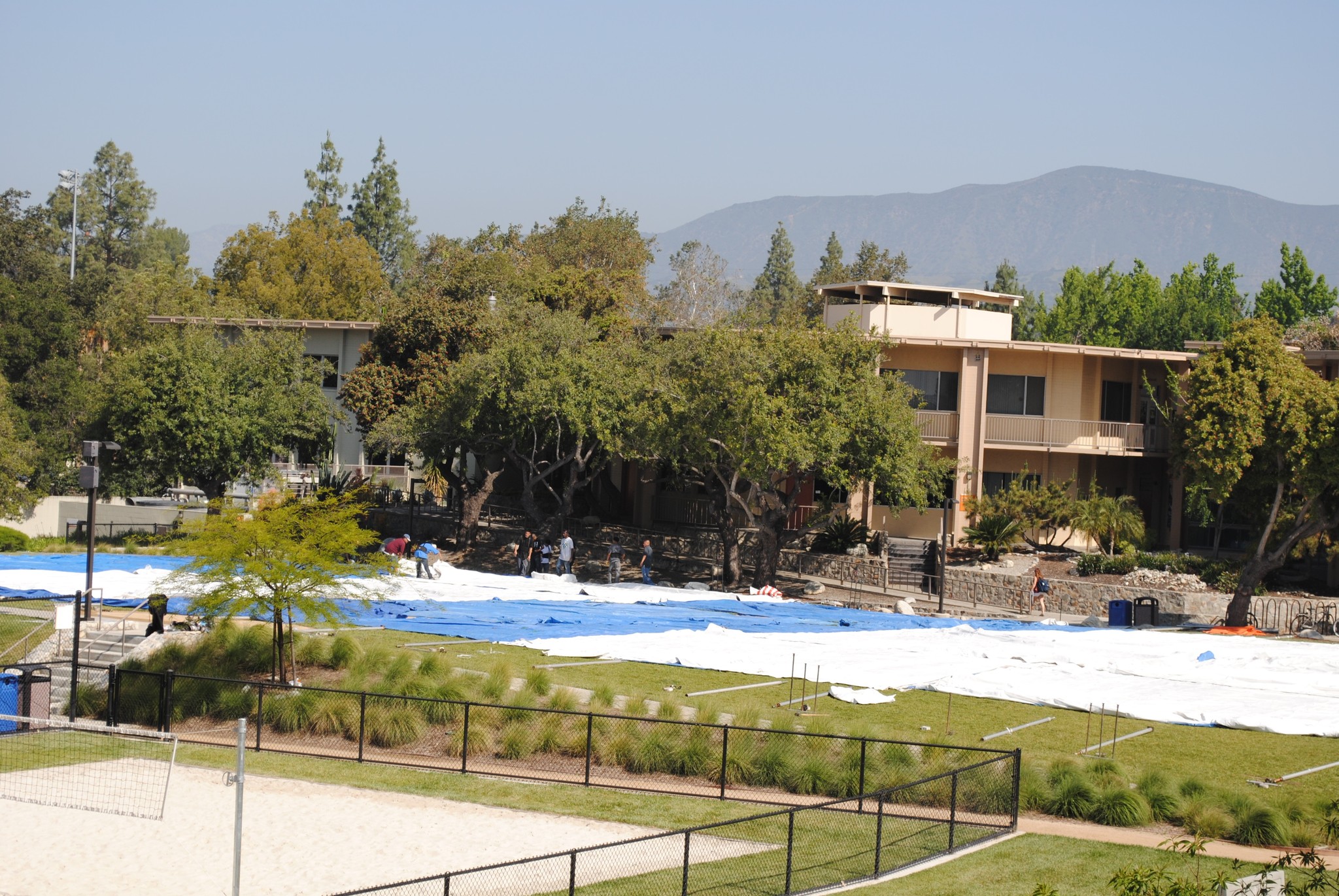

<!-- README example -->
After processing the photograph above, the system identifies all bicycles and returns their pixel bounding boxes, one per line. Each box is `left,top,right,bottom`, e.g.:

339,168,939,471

1290,605,1336,636
1333,618,1339,637
1220,597,1258,628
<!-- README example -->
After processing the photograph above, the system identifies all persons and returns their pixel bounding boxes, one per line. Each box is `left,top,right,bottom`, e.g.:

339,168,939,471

1025,568,1046,616
512,528,533,576
414,544,443,579
564,533,575,574
383,534,411,576
539,539,552,573
605,536,625,584
556,530,574,577
639,540,656,585
529,534,544,577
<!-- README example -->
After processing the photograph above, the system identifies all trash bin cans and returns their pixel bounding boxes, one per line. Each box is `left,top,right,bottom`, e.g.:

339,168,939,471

1108,600,1132,626
3,665,52,733
0,673,19,734
1134,597,1159,627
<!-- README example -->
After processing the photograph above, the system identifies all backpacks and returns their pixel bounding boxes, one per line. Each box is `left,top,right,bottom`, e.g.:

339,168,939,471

1037,578,1049,593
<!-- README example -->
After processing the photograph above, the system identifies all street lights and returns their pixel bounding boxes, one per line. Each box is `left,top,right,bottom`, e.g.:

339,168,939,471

79,439,122,619
935,498,960,613
57,169,81,290
406,478,427,560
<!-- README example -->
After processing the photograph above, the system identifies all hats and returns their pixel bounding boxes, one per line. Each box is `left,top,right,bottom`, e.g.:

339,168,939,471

563,529,570,536
404,534,411,541
